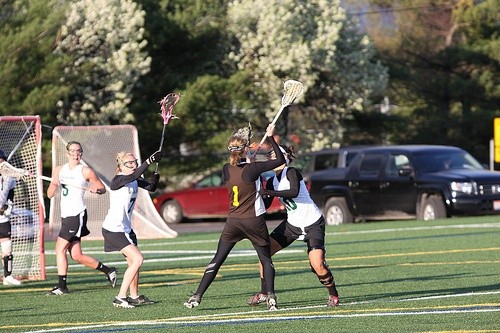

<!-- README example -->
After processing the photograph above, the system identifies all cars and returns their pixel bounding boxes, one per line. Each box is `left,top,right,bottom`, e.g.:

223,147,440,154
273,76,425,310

298,150,357,173
147,168,288,224
9,206,37,240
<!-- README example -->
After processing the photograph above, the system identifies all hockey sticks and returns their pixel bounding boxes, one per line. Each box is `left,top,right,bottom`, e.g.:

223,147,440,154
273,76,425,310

0,161,102,195
154,92,180,175
251,79,304,158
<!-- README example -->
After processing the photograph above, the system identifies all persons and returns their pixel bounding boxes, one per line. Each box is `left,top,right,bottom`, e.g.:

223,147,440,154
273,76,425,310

0,150,22,285
102,150,161,308
247,144,338,307
184,124,286,310
46,141,117,296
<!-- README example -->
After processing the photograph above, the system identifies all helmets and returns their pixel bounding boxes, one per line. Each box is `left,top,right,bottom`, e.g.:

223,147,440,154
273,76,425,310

0,149,7,161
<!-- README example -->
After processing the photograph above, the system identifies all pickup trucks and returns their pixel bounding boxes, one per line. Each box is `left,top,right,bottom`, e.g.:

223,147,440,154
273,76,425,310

308,145,500,226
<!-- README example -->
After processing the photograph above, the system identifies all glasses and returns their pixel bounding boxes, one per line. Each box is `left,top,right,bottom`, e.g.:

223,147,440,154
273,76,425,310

68,149,83,156
120,159,138,169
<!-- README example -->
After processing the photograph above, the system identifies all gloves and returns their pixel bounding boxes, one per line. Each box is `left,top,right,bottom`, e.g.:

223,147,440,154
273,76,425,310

260,189,272,197
151,171,160,186
4,199,13,217
147,150,162,165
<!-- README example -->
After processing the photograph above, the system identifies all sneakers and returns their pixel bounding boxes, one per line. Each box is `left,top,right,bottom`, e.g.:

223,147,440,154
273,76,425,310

328,294,338,306
183,293,201,309
113,296,135,308
45,287,69,296
3,275,21,285
107,267,117,289
126,295,156,306
247,293,267,306
266,294,277,310
